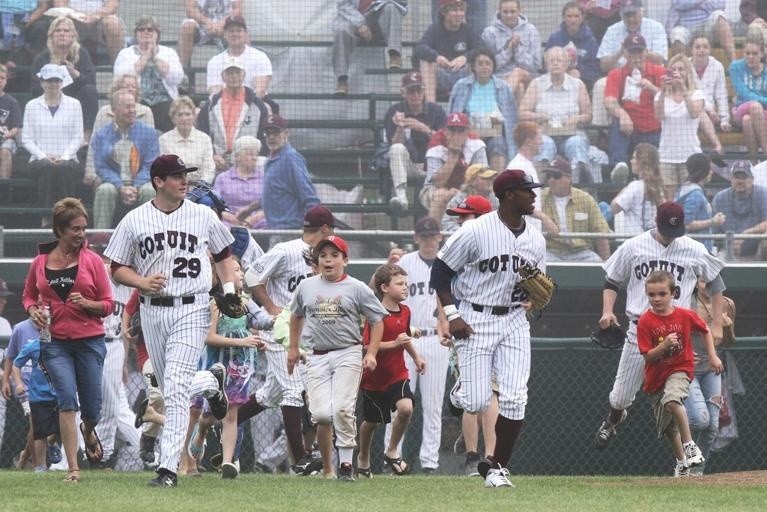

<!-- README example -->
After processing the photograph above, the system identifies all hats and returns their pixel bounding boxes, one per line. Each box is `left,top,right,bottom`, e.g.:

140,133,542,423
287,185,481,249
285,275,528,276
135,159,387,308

415,216,440,235
669,26,690,45
303,204,345,228
315,235,348,254
150,154,198,176
731,160,754,177
446,194,492,215
656,201,686,237
492,168,544,193
222,57,244,71
262,115,288,130
542,156,572,174
465,163,497,180
37,63,65,82
223,15,246,27
401,70,424,87
624,34,646,50
445,111,468,127
621,0,641,14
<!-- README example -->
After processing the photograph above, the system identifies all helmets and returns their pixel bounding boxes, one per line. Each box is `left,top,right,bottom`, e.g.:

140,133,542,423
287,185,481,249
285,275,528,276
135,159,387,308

187,182,225,207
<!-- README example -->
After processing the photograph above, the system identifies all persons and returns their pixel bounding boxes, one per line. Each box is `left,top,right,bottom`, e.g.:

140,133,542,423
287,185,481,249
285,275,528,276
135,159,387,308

190,257,267,484
119,286,168,466
94,91,162,228
668,0,739,61
652,53,705,199
418,114,489,223
687,37,732,154
479,0,544,101
368,218,451,479
599,33,666,156
605,142,667,240
380,68,449,214
96,262,134,470
673,152,725,257
244,207,344,476
196,55,268,169
159,97,216,185
517,45,591,153
84,71,155,186
437,191,500,477
3,294,58,470
546,1,601,83
179,0,244,70
412,0,487,103
208,133,266,229
0,276,18,457
112,14,184,131
261,115,323,234
286,236,384,483
593,201,725,452
428,168,547,488
184,184,263,265
505,119,559,237
594,0,669,73
0,64,22,180
328,0,408,94
356,262,427,478
730,35,766,157
445,163,503,232
0,1,49,49
539,158,612,267
187,186,262,274
21,64,85,227
22,198,115,482
52,0,126,61
739,1,767,38
684,274,736,475
106,154,241,488
12,331,61,475
30,16,93,122
713,159,767,259
444,48,515,157
636,271,725,480
206,14,272,101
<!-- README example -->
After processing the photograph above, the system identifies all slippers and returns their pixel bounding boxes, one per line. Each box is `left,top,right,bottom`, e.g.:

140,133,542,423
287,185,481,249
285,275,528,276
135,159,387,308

46,437,63,463
134,397,151,429
187,431,205,462
221,463,238,478
79,421,104,464
356,466,375,480
383,453,411,475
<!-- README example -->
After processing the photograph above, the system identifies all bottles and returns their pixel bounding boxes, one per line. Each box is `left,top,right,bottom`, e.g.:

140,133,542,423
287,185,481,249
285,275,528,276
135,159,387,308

39,306,51,342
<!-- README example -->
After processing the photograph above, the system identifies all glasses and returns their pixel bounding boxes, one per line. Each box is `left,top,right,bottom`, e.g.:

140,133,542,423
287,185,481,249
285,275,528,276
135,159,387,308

448,127,465,133
472,166,490,176
504,175,534,188
136,26,155,32
456,201,488,213
546,172,569,179
263,130,282,137
408,86,423,92
734,174,748,180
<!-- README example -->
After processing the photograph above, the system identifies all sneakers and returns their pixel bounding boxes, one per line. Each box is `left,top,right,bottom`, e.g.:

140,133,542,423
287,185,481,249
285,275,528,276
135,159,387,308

484,462,516,488
389,196,410,216
338,463,355,483
683,441,705,467
388,52,402,68
595,408,628,450
139,432,156,463
477,455,494,479
147,470,177,488
673,459,690,478
335,79,349,94
454,432,467,455
464,452,483,476
292,458,322,475
208,362,229,421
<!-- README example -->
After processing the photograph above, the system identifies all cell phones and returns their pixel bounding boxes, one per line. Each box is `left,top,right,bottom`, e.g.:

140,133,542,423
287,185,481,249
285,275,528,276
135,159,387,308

664,68,674,79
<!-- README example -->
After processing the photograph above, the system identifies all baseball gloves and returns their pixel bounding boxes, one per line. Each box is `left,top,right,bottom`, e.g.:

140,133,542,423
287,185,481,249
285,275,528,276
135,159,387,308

518,261,556,312
591,322,626,350
213,291,258,322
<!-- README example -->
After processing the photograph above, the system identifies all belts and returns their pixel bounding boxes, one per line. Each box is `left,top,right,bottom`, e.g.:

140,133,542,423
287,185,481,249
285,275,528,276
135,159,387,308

421,329,438,336
138,294,195,307
471,303,521,315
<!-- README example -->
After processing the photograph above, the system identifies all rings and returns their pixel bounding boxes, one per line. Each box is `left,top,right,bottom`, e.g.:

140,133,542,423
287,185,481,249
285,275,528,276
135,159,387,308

73,299,77,304
